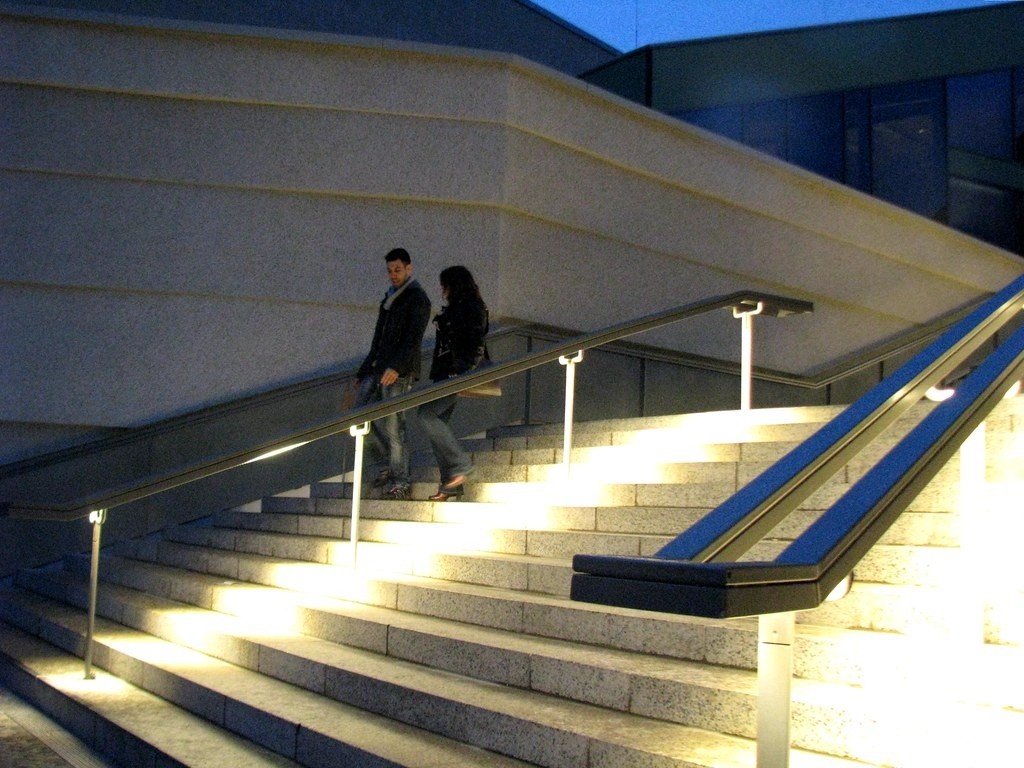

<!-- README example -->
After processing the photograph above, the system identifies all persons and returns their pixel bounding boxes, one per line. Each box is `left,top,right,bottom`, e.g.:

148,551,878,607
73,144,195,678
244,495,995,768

416,266,490,501
350,247,432,500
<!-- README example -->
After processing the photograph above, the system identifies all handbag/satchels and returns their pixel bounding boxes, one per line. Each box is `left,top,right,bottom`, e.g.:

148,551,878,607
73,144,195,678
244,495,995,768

456,372,502,400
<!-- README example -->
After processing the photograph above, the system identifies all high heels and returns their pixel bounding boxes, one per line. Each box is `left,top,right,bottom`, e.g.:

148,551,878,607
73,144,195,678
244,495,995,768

429,491,462,502
445,469,468,488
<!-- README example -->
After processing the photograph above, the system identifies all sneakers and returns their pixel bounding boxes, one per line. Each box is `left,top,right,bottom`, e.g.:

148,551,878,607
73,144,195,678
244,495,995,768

385,488,412,501
374,459,393,479
